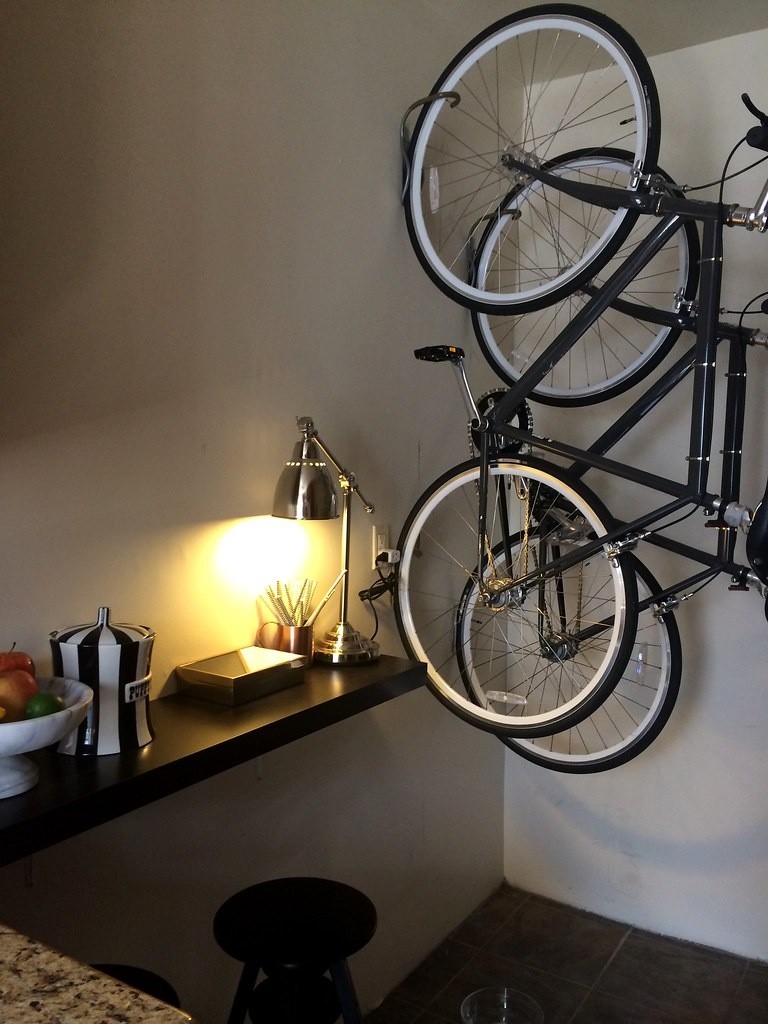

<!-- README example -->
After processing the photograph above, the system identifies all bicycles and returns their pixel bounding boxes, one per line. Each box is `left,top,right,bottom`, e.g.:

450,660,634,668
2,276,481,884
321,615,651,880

402,1,767,775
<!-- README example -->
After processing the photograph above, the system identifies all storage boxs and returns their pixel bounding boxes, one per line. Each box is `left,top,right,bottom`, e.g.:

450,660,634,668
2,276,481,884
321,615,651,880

50,606,156,755
176,645,307,708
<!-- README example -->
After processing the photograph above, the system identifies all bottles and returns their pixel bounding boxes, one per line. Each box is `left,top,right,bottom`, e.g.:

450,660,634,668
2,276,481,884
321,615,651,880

49,607,155,757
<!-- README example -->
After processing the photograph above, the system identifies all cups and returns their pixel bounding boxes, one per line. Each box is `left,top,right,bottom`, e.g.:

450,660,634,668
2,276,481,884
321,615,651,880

460,986,545,1024
256,620,314,669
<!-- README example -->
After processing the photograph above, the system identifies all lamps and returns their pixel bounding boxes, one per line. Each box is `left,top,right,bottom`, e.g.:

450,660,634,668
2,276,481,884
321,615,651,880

272,414,380,666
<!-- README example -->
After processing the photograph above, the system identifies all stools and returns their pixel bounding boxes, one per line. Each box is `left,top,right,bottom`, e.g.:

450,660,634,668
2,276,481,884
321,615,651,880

90,965,181,1011
214,876,377,1024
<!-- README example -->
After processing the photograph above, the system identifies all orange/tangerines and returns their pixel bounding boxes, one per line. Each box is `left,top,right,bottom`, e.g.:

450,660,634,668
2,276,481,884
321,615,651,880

26,693,65,719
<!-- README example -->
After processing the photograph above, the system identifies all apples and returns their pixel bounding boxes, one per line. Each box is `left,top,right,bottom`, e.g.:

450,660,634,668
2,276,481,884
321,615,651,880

0,642,36,678
0,670,38,721
